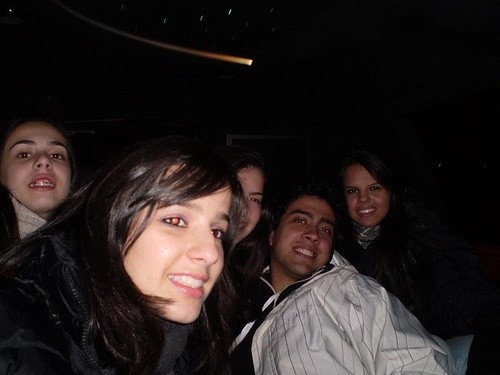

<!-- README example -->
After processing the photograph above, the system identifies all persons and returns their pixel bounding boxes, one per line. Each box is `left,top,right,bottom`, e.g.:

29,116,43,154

320,150,499,375
205,143,279,372
229,181,456,375
0,114,79,264
0,133,246,375
